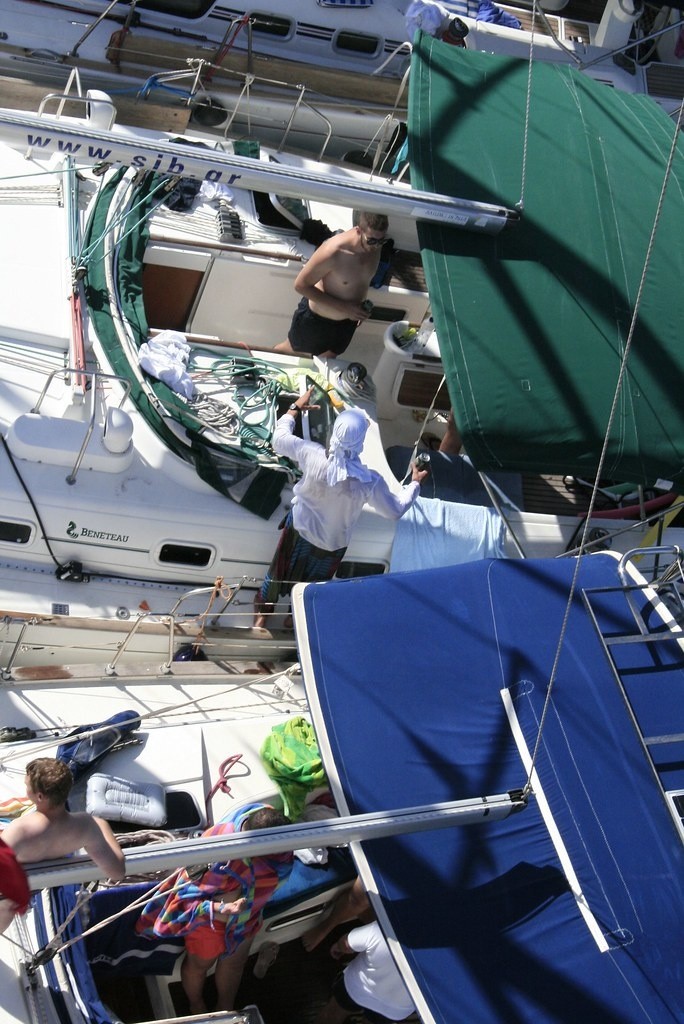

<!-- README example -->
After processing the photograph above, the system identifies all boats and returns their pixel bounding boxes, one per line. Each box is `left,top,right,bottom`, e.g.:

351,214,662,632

0,0,684,1024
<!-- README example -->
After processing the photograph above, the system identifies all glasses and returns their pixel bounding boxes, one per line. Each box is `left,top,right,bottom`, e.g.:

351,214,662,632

357,226,385,245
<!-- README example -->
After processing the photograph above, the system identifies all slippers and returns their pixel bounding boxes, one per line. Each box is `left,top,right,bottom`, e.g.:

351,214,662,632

252,940,280,979
421,432,442,451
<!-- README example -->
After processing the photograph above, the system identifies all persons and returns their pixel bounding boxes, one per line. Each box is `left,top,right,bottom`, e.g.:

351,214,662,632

313,920,416,1024
302,875,372,952
133,803,293,1015
274,211,388,359
0,758,125,881
253,384,427,627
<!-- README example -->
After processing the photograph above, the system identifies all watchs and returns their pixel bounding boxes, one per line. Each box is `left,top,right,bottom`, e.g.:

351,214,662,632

289,403,300,414
